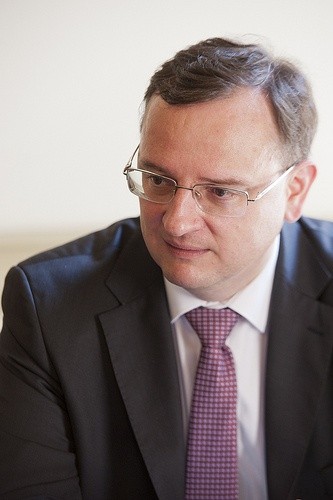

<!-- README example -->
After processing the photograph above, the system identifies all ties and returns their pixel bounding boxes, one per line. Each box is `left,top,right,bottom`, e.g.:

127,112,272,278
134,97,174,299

183,307,239,500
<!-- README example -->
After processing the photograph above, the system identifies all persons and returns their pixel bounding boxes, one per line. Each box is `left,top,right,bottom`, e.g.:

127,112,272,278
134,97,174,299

0,37,332,500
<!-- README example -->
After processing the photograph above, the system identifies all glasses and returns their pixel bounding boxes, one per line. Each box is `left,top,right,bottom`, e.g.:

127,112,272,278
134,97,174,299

123,144,299,217
